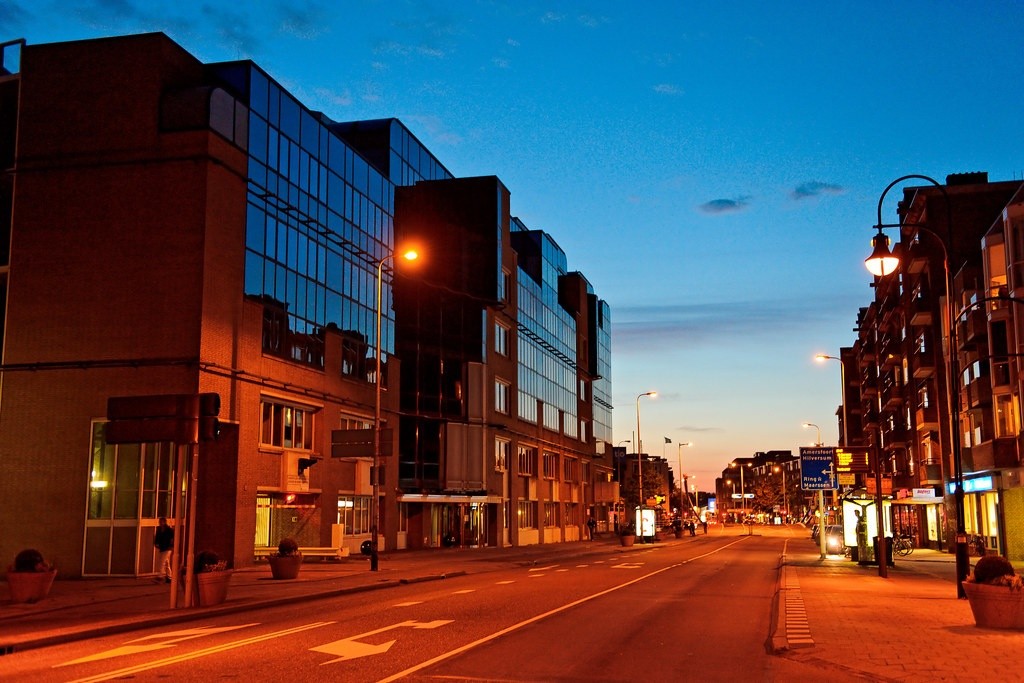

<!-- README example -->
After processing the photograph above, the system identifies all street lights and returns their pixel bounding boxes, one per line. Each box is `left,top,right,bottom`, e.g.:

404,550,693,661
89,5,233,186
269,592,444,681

861,173,972,601
692,485,699,528
807,422,840,524
731,462,753,510
617,439,631,537
370,249,422,570
677,442,692,531
774,465,786,524
635,391,657,544
727,480,736,509
815,354,851,494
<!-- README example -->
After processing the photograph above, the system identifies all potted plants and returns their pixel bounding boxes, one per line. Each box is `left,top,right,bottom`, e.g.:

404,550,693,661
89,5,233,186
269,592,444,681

620,526,637,546
674,526,684,538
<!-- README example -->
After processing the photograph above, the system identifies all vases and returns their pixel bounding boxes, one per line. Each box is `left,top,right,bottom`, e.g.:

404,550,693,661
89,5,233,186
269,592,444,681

9,570,58,603
180,570,233,606
962,581,1024,629
270,555,302,579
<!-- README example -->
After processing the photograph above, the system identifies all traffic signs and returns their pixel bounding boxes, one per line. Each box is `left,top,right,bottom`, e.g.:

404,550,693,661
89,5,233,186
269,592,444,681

798,445,839,491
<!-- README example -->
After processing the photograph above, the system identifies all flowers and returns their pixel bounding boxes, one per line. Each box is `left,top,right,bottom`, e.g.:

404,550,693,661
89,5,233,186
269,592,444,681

965,554,1024,590
269,538,302,558
5,549,53,572
183,551,233,573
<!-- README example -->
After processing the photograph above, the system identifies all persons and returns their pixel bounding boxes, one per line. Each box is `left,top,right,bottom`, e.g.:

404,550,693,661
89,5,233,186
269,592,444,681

854,510,868,565
154,517,174,584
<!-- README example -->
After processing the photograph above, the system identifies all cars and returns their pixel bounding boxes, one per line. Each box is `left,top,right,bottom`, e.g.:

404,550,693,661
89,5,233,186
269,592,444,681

812,522,852,558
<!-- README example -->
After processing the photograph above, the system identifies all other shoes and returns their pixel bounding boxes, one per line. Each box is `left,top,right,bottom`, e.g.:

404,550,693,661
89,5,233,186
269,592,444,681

165,578,171,584
152,578,161,584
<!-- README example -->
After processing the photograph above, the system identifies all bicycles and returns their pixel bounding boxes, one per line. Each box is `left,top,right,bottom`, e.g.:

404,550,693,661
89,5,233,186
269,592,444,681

892,528,916,557
